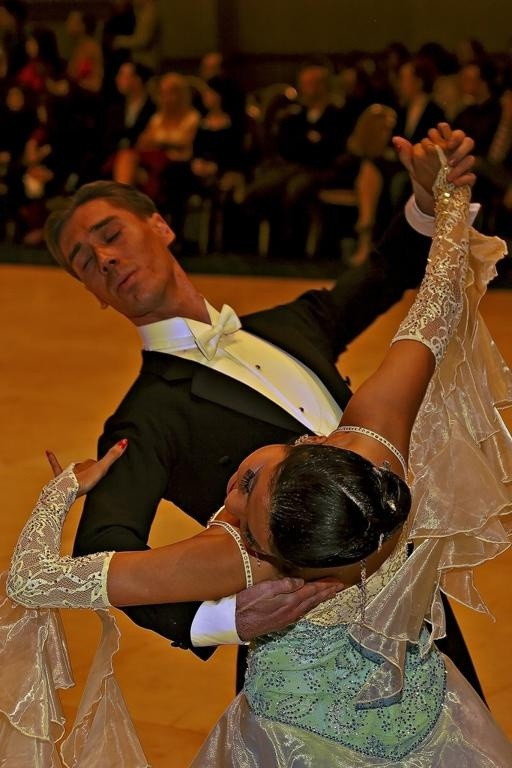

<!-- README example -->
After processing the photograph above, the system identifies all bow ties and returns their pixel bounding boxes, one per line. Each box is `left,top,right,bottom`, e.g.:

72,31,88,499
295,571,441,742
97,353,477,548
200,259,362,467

194,304,242,362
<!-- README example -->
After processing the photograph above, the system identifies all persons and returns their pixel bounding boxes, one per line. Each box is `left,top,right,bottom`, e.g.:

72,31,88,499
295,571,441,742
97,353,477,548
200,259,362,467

43,122,490,710
1,0,511,268
0,122,512,768
